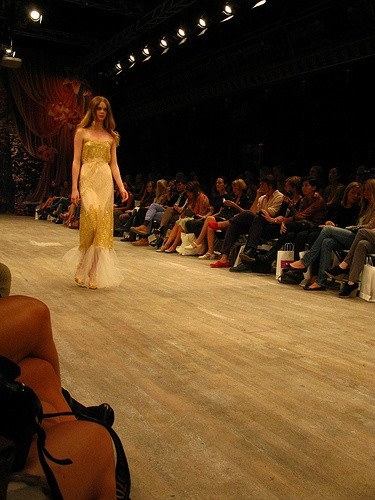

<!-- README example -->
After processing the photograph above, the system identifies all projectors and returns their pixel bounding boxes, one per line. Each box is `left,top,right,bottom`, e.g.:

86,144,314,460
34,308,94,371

0,57,22,68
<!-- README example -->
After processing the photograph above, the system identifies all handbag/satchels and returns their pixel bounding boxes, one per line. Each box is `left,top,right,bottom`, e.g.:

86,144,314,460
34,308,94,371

359,256,375,303
275,242,311,284
176,232,196,256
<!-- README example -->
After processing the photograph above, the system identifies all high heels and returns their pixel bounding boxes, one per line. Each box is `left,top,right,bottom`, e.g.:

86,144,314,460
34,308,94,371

324,265,347,281
338,282,359,298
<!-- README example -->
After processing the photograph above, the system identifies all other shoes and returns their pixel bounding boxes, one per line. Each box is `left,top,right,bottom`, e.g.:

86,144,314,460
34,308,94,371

130,225,148,234
207,221,222,230
73,277,87,288
240,249,256,262
131,238,150,246
229,263,250,272
184,242,202,250
285,262,308,273
88,270,98,289
155,248,165,252
164,248,175,252
148,239,164,249
210,260,230,267
198,251,215,260
303,284,326,291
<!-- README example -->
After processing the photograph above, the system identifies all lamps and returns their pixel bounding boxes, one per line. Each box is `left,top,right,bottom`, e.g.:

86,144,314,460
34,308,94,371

112,0,266,78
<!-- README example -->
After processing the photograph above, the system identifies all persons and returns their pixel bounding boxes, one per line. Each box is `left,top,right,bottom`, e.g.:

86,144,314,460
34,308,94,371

0,263,117,500
36,180,80,229
113,166,375,298
71,96,129,290
